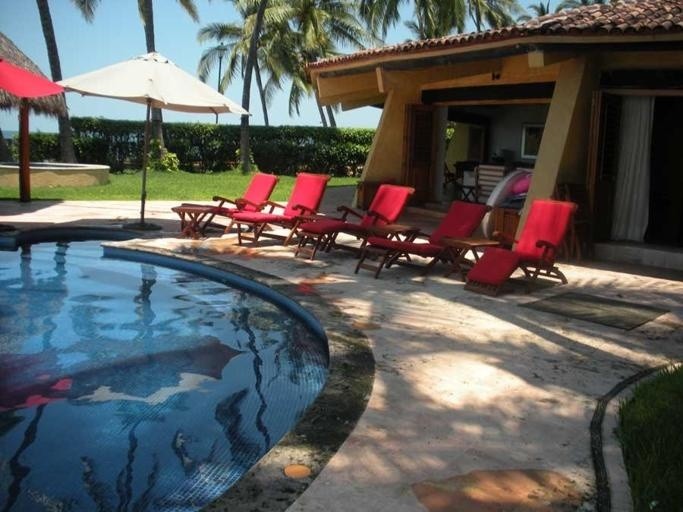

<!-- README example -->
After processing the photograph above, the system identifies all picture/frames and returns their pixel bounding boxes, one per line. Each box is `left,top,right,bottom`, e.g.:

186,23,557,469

520,122,545,160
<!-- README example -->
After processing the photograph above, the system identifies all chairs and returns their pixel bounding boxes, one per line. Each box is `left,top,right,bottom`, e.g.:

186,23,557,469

452,157,484,204
227,171,330,246
465,199,579,297
295,183,414,260
354,203,493,280
171,172,284,238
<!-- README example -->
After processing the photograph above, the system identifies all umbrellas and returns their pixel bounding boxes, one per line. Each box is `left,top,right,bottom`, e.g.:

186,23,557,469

0,60,65,103
53,50,255,226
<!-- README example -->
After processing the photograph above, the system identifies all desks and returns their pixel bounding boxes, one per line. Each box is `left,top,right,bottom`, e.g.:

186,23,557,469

366,223,421,262
444,236,500,281
